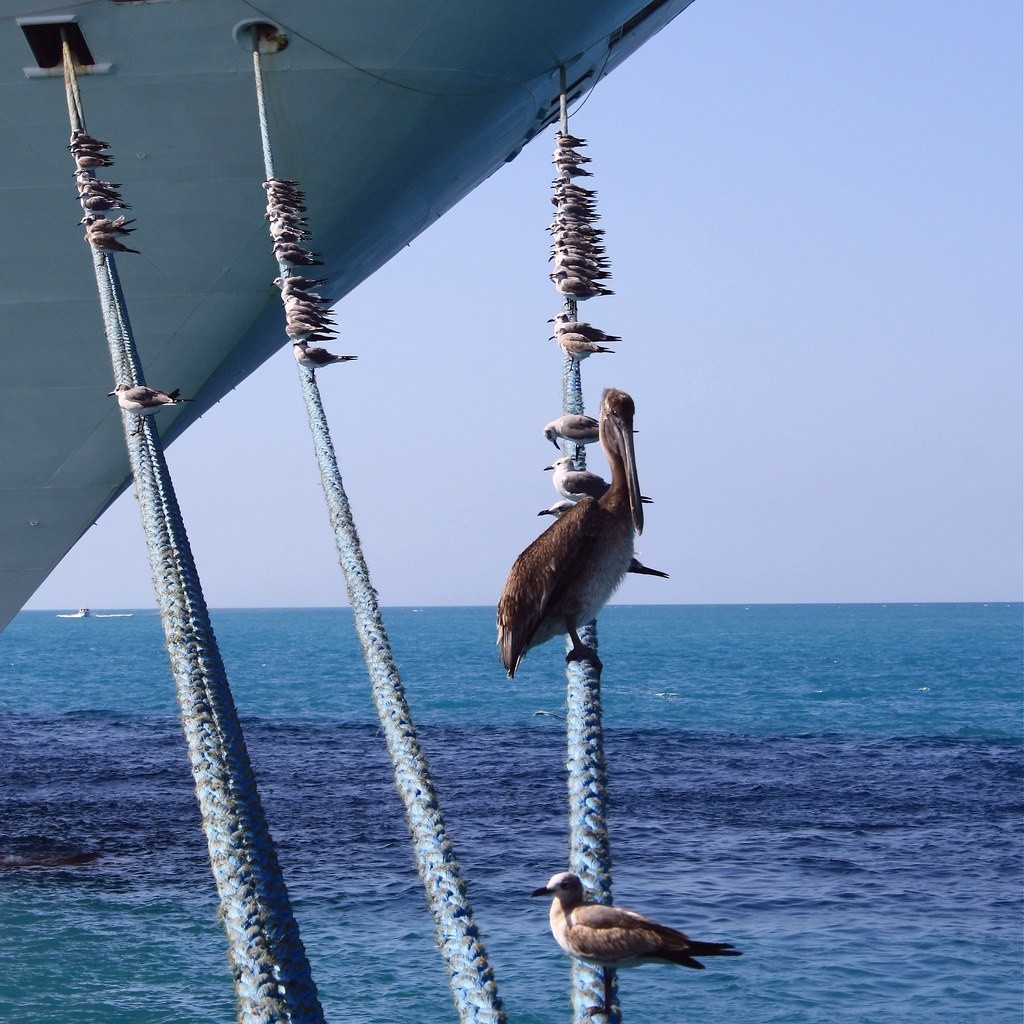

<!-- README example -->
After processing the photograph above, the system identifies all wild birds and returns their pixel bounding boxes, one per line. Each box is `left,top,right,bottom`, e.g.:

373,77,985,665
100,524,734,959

66,127,671,583
530,872,745,1015
109,383,181,437
495,389,644,679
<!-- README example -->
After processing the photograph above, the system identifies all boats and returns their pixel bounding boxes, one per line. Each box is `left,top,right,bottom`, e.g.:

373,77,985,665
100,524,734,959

79,606,90,619
